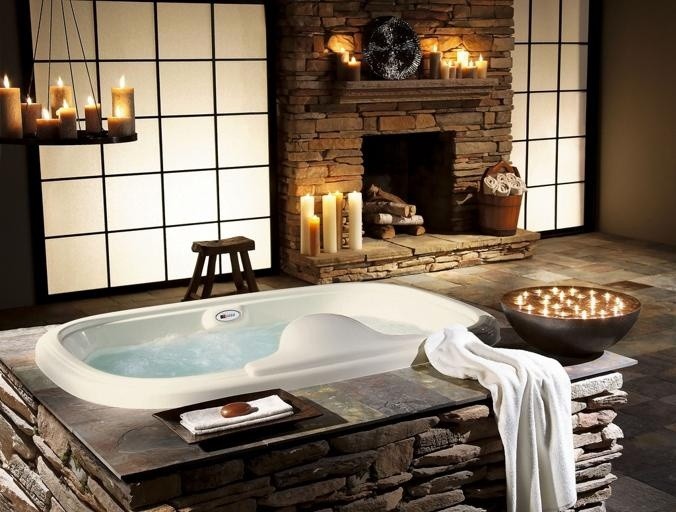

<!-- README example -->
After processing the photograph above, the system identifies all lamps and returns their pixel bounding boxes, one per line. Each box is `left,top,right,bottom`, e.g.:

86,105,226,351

1,0,137,146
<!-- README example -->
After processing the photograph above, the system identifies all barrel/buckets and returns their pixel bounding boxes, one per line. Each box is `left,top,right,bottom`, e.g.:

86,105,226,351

481,158,525,235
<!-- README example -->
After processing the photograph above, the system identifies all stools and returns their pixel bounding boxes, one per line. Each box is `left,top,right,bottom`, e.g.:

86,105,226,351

180,235,260,301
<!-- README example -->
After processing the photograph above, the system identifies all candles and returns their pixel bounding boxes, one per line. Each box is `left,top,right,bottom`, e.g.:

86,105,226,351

429,50,489,79
298,190,366,257
335,50,363,79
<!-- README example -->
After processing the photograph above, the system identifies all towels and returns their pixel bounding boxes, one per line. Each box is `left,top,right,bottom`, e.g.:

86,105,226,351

483,172,528,197
178,394,295,437
423,323,578,512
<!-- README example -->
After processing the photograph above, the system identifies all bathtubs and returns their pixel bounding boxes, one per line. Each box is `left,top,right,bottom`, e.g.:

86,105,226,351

34,277,501,408
